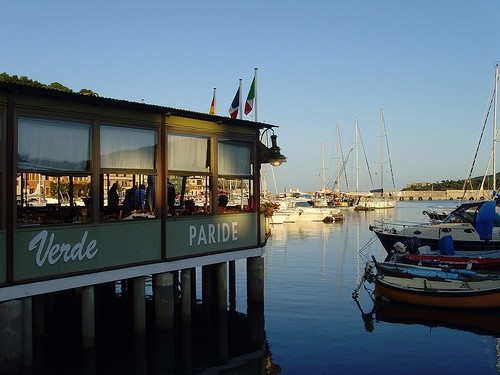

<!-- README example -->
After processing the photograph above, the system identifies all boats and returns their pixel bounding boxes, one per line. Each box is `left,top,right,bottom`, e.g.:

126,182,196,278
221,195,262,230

368,189,500,256
266,197,344,223
352,255,500,311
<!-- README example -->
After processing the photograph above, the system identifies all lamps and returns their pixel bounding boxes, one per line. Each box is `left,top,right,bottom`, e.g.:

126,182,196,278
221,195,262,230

261,127,288,168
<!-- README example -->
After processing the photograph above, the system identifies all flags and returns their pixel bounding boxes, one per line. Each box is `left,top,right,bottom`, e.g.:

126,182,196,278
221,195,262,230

244,73,256,115
209,90,216,115
228,86,240,120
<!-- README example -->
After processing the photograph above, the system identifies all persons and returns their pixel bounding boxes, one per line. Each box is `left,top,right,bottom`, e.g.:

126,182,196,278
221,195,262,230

107,182,119,211
168,177,177,212
122,182,148,217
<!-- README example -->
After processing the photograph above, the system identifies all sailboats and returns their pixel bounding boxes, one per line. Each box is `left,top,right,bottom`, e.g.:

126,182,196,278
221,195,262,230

261,113,374,208
422,60,499,222
352,107,397,211
